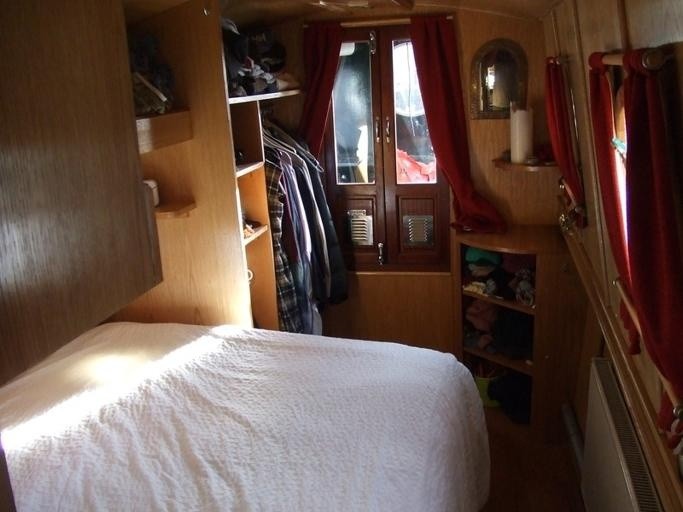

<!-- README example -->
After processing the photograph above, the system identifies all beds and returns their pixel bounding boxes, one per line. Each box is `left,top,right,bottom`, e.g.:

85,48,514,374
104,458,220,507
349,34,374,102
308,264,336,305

0,321,491,512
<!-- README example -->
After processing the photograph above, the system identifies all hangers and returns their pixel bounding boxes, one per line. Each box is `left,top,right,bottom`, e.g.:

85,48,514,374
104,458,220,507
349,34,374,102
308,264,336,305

260,109,313,157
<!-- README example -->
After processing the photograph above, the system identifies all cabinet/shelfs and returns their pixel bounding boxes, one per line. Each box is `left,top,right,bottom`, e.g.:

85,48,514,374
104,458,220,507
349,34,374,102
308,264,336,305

0,0,163,390
136,107,196,220
449,226,590,432
104,1,304,387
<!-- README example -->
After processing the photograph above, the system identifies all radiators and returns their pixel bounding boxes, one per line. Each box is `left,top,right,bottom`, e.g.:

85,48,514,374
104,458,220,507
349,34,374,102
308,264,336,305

562,356,664,512
